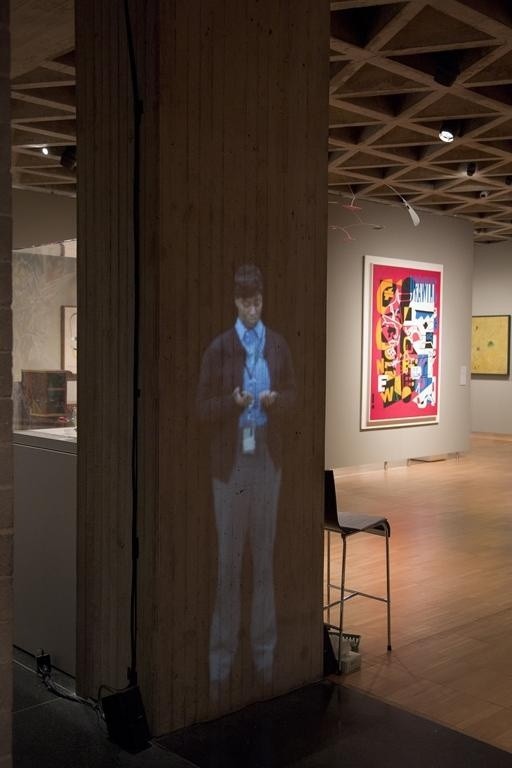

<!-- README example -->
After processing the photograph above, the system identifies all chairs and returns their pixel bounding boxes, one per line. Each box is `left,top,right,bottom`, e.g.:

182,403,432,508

325,466,394,684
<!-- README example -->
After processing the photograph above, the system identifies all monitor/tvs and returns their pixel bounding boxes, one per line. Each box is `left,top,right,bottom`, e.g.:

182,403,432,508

21,369,67,430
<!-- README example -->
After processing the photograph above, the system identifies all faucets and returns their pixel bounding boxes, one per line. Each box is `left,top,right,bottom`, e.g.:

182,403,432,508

70,406,77,429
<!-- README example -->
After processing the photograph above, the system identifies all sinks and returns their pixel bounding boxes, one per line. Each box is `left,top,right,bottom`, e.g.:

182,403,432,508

29,427,77,438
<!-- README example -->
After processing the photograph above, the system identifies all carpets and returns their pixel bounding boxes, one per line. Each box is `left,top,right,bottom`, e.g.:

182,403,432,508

153,675,512,765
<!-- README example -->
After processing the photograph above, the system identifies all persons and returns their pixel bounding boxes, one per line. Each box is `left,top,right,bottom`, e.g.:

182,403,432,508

195,261,303,703
